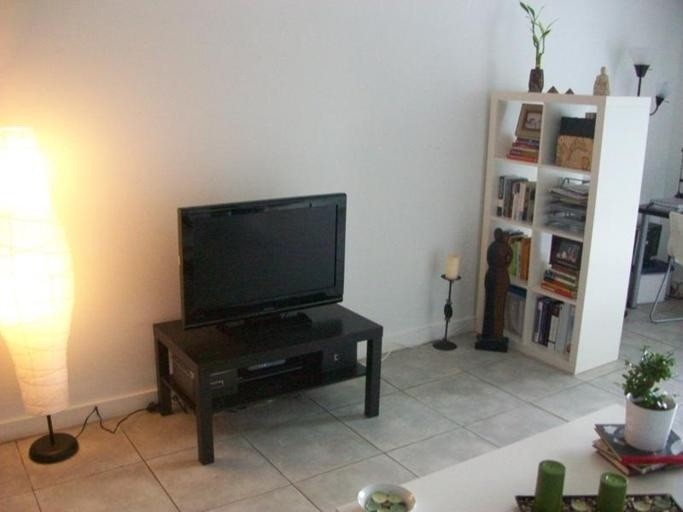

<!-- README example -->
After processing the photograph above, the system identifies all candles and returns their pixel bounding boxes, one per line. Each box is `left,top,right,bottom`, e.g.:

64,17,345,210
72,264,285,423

532,458,627,511
444,255,460,280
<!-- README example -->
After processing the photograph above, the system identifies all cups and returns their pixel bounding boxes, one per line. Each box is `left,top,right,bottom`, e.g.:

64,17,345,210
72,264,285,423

594,472,628,512
532,458,566,512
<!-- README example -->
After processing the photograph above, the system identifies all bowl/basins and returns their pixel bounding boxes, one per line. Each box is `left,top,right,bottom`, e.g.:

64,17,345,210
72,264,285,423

356,481,417,511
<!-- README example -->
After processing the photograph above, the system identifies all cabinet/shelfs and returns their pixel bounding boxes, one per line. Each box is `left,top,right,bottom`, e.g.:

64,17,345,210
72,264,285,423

474,89,652,376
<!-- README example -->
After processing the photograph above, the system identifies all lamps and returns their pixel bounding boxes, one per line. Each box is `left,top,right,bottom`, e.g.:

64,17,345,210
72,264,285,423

630,44,669,115
0,127,80,464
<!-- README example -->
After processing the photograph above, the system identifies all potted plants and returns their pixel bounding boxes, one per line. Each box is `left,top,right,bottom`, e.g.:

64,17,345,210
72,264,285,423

621,344,678,451
519,1,558,93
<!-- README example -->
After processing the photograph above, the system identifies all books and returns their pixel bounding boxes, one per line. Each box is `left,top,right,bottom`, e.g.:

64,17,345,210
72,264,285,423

589,419,683,479
494,174,594,362
506,136,539,163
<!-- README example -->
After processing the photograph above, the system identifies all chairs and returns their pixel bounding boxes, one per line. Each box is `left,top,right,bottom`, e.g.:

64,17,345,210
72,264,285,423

649,210,683,324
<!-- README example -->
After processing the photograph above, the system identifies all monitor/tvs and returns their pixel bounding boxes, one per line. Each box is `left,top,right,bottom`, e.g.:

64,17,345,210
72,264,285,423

178,193,346,338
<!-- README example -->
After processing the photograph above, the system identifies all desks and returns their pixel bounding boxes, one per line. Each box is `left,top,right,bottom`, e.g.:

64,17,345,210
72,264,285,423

153,303,383,467
335,401,683,512
629,199,683,309
